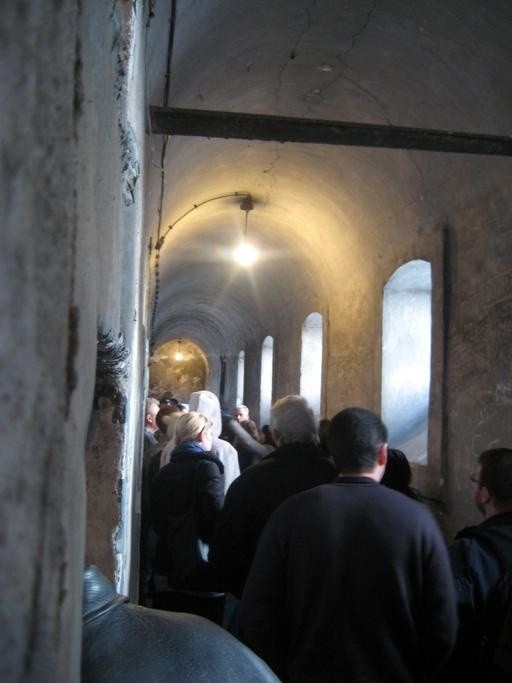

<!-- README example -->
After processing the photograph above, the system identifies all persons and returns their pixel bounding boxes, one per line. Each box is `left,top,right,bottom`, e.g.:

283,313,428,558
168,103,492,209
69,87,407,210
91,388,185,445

81,333,278,683
142,390,512,683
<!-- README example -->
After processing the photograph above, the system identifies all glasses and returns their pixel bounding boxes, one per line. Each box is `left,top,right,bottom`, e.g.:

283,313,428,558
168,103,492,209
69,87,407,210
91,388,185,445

470,473,480,486
